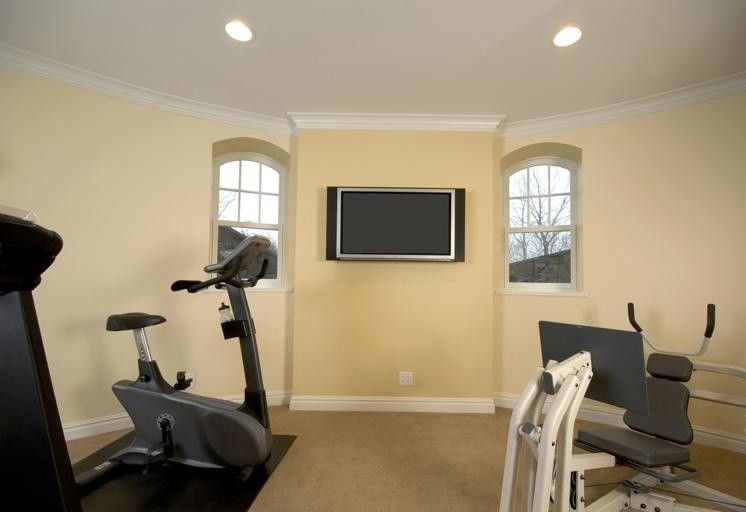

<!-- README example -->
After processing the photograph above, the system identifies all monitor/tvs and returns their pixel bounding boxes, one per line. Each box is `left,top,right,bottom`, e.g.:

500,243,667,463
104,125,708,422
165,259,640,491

538,320,653,418
203,234,271,273
325,185,466,263
